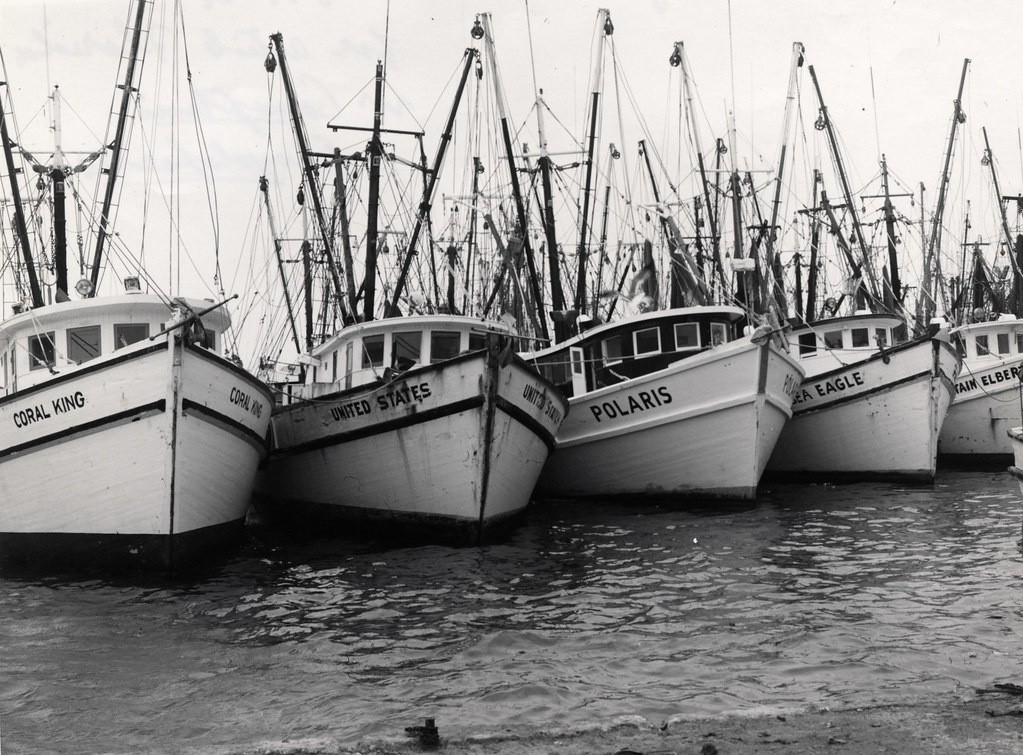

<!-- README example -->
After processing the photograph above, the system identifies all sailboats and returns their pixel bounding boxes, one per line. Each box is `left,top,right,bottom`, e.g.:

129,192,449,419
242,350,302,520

691,2,967,491
0,0,281,598
214,2,573,545
467,2,811,519
887,52,1023,486
